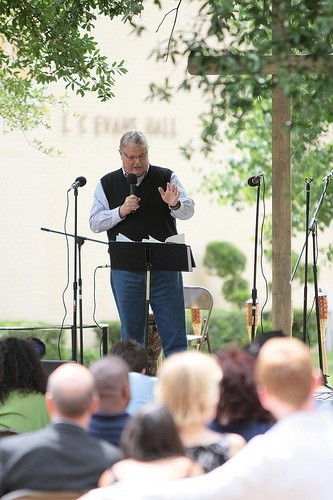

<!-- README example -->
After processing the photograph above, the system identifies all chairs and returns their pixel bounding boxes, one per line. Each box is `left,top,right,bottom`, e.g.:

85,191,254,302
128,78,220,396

183,286,214,354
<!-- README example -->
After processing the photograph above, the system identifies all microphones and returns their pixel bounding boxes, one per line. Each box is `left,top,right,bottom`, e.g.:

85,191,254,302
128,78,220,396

71,176,86,189
127,174,137,214
248,174,263,187
322,170,333,181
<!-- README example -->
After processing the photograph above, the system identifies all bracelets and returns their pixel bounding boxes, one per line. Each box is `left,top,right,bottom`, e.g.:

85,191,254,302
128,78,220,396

170,200,181,211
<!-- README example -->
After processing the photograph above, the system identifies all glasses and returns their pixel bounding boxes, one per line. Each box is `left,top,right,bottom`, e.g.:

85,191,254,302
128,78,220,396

120,149,148,160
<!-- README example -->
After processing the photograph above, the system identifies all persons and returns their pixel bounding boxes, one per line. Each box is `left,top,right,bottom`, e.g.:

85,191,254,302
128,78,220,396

0,337,333,500
88,130,196,359
